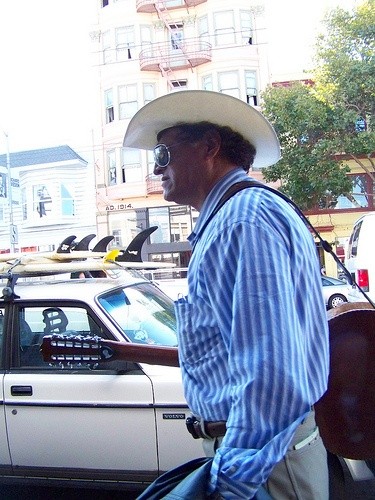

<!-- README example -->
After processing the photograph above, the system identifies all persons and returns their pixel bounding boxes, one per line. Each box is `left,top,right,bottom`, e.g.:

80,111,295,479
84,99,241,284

122,85,332,500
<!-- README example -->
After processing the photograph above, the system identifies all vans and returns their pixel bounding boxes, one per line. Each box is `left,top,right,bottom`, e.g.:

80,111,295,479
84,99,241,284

344,211,375,303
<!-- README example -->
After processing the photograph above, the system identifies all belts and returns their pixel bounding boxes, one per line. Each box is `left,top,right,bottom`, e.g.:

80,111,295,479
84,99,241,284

185,417,227,440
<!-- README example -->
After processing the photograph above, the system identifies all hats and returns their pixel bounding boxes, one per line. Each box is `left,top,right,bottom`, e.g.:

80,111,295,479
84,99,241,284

122,90,282,169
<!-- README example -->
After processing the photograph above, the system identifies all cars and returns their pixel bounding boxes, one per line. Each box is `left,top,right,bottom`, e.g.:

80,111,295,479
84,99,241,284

321,275,348,309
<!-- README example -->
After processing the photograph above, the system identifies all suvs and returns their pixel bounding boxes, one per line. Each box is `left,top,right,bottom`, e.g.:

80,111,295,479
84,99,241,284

0,226,206,490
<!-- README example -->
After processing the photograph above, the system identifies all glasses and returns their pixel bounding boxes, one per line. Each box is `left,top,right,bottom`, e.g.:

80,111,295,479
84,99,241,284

153,139,199,168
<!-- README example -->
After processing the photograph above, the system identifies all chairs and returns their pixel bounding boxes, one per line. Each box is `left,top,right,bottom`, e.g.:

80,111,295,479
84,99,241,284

43,308,69,339
0,317,39,366
